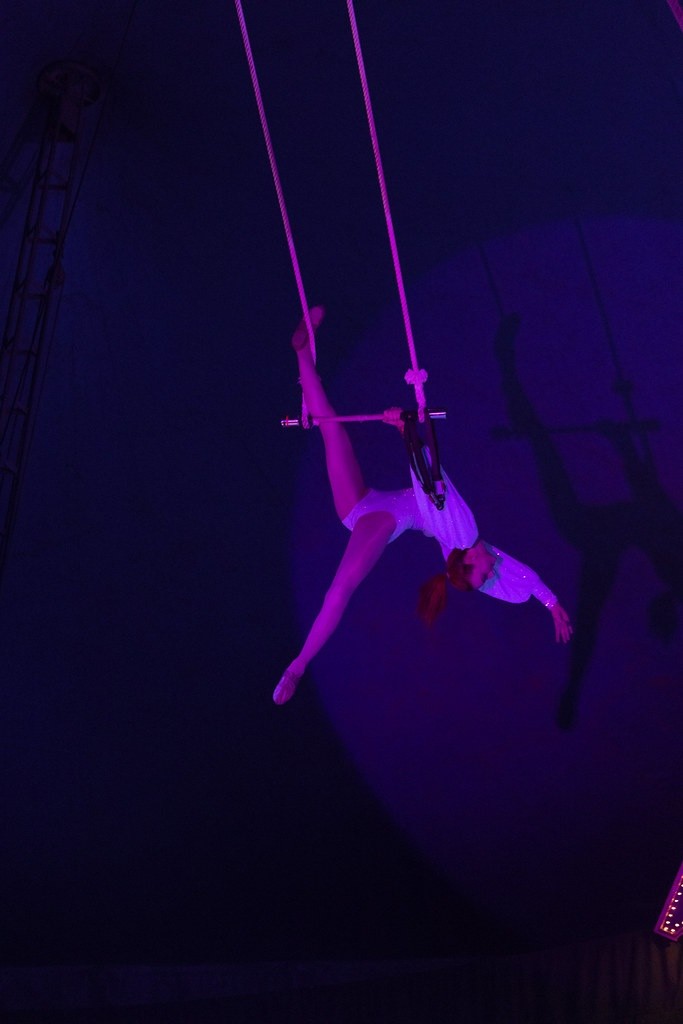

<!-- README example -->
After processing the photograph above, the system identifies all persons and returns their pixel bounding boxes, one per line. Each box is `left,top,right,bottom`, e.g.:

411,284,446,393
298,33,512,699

271,304,574,708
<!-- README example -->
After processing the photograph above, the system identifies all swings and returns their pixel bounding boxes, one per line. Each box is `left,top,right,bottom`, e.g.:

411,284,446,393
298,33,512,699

236,0,450,429
